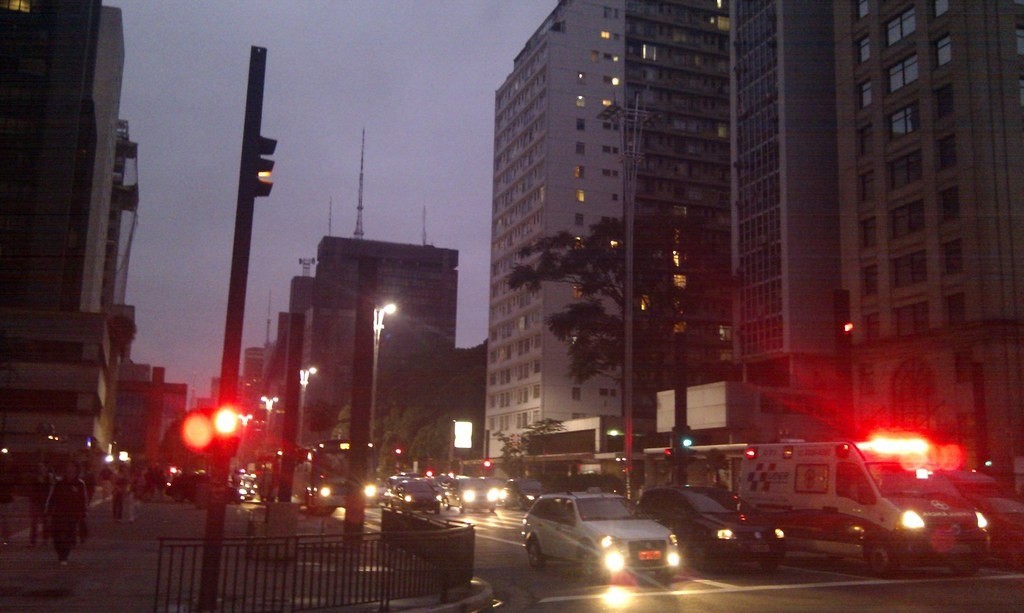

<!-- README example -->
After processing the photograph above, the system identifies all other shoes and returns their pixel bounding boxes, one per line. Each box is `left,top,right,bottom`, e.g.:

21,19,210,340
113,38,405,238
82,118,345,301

129,518,135,522
59,560,68,565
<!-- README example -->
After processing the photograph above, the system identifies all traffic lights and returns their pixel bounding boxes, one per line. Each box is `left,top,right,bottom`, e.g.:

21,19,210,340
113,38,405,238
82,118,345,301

253,137,280,198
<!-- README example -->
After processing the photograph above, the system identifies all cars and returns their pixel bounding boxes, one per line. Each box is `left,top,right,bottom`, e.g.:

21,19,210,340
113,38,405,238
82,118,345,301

239,468,548,514
940,468,1024,570
639,486,784,572
521,490,682,585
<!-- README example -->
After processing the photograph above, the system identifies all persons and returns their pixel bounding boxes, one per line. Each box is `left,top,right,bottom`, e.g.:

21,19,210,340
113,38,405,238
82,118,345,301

27,458,149,565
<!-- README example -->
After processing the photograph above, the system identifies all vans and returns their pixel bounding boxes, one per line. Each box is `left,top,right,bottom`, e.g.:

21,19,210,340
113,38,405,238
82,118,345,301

739,439,990,574
